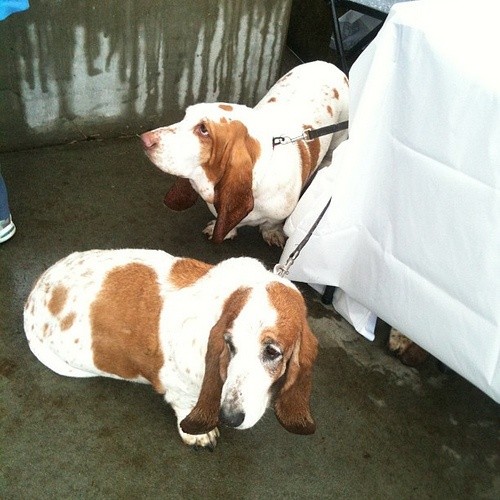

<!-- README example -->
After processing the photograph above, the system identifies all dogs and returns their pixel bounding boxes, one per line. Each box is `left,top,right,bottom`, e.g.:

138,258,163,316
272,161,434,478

21,247,319,451
138,60,350,248
